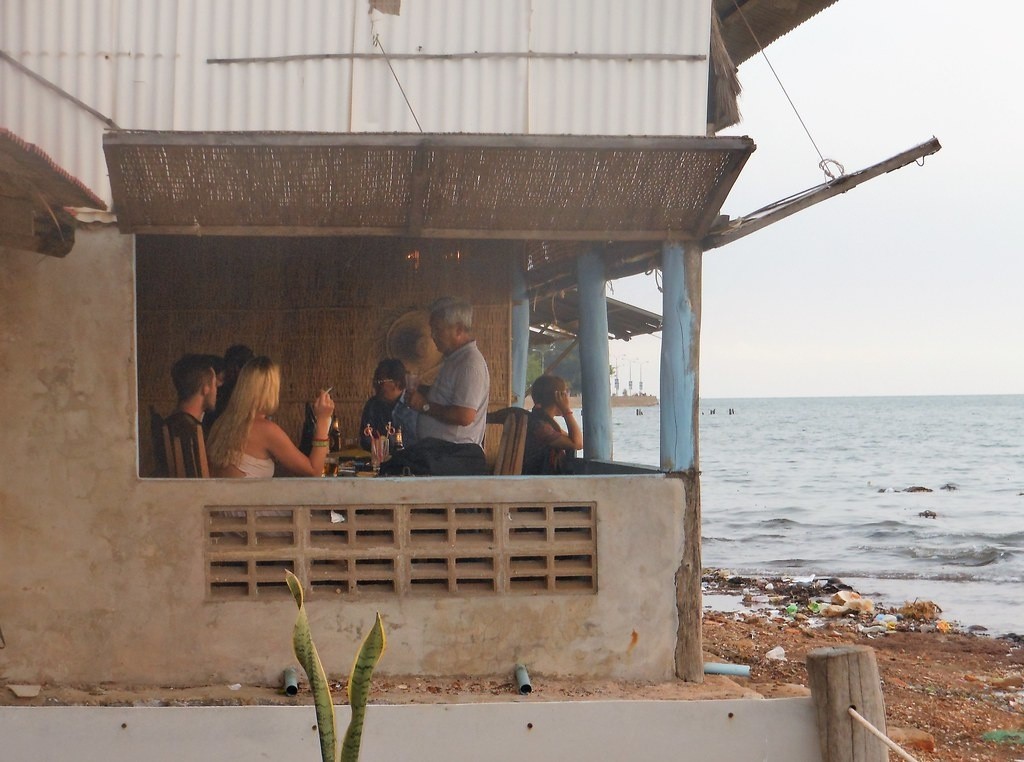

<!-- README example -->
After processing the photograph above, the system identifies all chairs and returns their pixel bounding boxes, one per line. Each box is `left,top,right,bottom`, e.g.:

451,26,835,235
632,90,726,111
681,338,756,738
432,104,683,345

149,406,222,539
481,407,535,513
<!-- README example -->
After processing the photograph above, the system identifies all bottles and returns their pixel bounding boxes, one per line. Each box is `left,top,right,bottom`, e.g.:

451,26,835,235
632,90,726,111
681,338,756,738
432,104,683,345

329,418,341,452
810,602,820,613
786,605,797,614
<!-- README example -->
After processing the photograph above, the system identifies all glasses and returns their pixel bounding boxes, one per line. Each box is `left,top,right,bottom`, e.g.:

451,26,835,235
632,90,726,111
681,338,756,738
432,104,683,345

373,379,395,386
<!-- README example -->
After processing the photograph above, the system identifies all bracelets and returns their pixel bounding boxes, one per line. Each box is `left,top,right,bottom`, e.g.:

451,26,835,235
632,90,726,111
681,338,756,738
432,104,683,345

311,437,330,447
562,410,573,417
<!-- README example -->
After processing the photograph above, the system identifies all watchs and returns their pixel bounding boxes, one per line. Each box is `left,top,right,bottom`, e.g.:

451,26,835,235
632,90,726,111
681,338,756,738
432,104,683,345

422,402,431,415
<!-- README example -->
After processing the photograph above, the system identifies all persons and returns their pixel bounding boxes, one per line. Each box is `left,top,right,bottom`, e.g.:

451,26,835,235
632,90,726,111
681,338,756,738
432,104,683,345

359,358,418,452
163,343,254,478
204,352,350,587
521,372,584,580
405,296,491,584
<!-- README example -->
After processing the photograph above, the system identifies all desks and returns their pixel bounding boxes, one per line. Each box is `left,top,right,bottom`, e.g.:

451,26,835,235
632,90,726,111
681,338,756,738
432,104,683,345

280,448,377,477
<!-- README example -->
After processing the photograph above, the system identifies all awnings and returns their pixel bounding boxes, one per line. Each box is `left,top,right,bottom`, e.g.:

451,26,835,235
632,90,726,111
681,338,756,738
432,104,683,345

529,287,662,345
100,131,941,281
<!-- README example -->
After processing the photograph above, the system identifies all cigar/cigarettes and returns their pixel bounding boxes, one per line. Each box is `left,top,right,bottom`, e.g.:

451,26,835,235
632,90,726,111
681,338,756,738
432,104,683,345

325,387,332,394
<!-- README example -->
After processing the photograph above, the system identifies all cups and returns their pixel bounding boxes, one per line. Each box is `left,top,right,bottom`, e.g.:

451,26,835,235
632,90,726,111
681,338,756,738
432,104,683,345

371,439,389,475
